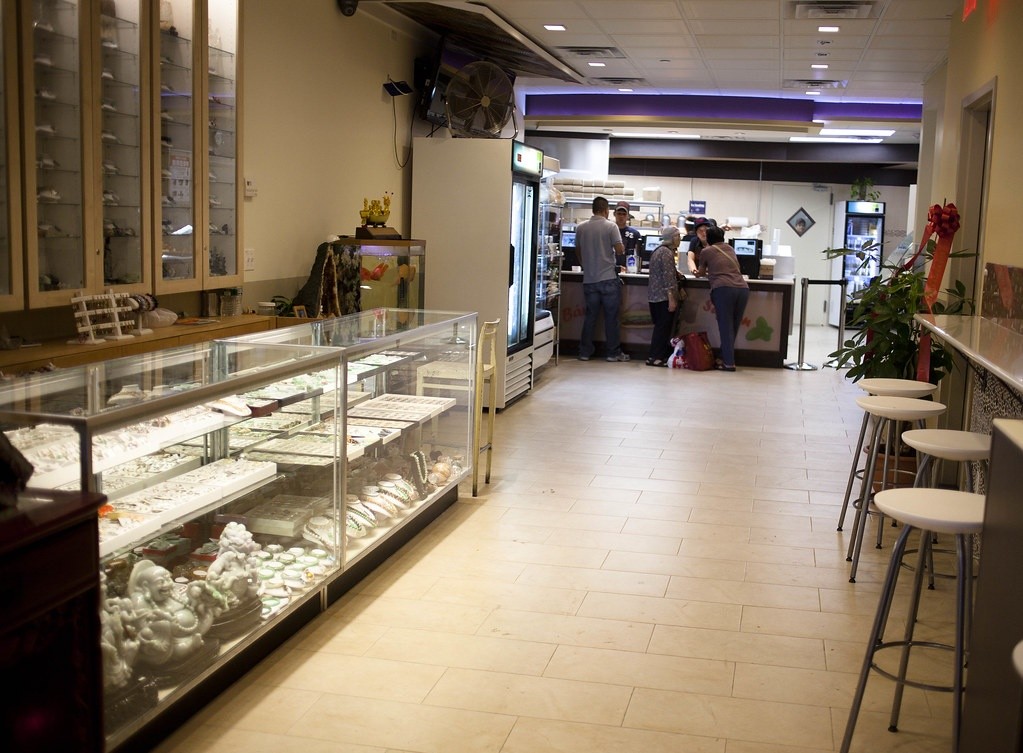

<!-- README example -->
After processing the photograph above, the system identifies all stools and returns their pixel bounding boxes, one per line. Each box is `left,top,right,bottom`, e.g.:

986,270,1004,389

414,318,501,497
836,377,992,753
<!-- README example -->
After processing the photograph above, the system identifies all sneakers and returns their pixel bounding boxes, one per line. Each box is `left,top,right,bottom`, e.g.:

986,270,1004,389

607,352,630,361
580,355,589,360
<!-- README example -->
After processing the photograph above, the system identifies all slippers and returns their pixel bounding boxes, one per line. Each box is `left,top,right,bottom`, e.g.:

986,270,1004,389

715,359,723,365
716,363,736,371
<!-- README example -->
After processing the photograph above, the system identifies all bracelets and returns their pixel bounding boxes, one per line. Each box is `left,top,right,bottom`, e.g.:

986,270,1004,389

667,292,674,295
132,294,159,313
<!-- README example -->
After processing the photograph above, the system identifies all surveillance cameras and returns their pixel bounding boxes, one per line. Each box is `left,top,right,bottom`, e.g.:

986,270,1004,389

337,0,359,17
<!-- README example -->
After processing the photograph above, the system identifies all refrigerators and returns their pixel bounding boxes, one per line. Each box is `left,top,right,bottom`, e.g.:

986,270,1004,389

408,138,545,411
829,201,886,328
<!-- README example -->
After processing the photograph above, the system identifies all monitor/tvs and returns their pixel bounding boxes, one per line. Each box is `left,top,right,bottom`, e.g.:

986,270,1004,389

420,42,517,138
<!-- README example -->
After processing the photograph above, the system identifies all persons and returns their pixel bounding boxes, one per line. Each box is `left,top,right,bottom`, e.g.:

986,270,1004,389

687,216,714,277
574,196,632,362
613,201,643,268
694,226,749,372
645,226,688,368
795,217,806,233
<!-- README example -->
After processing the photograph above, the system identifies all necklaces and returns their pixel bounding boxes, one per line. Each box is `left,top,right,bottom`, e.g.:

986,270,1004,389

0,312,468,738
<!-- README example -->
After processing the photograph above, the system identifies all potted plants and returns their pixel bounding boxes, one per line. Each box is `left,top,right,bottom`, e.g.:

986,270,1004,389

818,237,975,495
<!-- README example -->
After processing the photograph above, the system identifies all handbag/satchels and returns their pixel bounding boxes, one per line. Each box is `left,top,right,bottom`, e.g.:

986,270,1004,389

667,337,688,369
685,330,715,370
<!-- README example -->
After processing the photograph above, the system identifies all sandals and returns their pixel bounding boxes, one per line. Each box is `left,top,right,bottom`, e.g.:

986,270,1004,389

646,355,668,367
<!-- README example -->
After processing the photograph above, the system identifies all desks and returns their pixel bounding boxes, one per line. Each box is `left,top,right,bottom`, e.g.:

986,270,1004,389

913,312,1023,394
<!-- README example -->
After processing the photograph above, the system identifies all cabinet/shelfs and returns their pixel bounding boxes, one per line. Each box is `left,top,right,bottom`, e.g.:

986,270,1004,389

0,309,479,753
564,198,665,235
0,0,278,377
539,204,564,366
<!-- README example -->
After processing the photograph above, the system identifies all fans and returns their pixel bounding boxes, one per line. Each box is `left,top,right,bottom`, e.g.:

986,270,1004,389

446,60,519,140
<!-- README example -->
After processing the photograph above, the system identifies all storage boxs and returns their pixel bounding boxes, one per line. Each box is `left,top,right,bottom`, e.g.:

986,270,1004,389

330,238,426,340
575,218,662,228
643,189,661,202
760,265,774,280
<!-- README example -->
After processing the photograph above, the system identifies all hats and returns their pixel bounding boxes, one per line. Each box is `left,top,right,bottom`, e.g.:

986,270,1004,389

615,201,629,213
693,217,711,232
796,218,805,224
